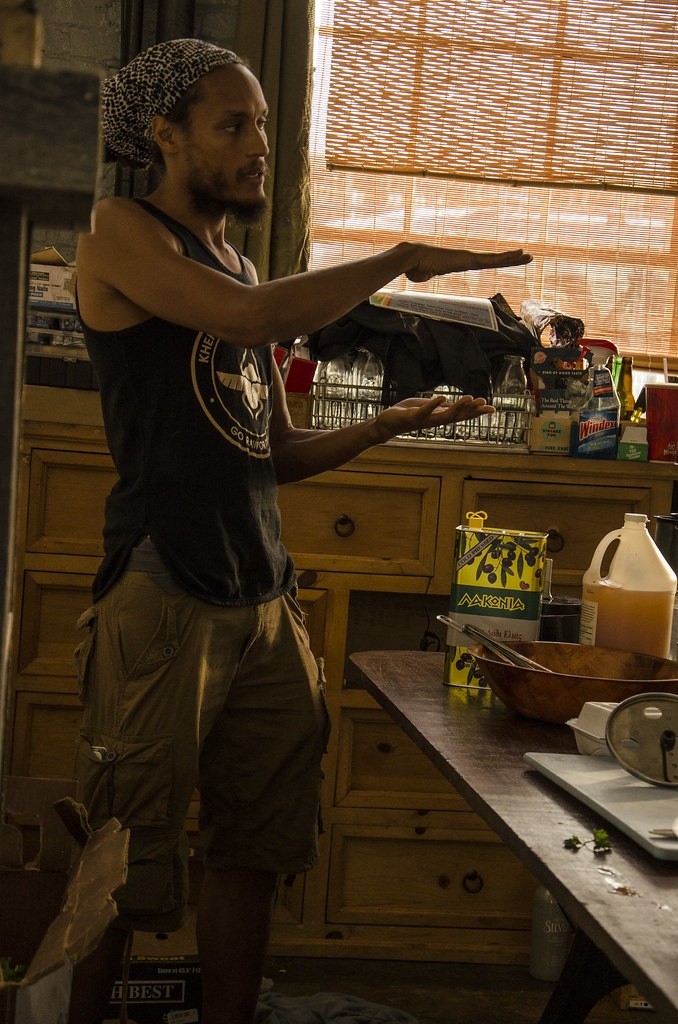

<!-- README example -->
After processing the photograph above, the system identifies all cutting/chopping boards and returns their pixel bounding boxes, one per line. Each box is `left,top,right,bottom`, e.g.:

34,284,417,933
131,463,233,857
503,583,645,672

524,751,678,859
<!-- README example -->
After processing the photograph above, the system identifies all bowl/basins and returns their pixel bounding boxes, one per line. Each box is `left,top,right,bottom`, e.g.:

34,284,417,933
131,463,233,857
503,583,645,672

466,641,678,723
567,701,621,756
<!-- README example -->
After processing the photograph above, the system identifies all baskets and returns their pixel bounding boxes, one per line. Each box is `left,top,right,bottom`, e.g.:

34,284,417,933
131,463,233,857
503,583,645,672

306,381,536,446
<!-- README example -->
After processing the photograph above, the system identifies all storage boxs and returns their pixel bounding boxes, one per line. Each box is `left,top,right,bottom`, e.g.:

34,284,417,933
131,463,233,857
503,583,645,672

616,425,649,461
273,346,290,369
285,358,317,393
27,263,77,312
0,797,130,1024
531,386,572,416
282,345,310,383
104,954,201,1024
525,416,572,455
286,391,312,429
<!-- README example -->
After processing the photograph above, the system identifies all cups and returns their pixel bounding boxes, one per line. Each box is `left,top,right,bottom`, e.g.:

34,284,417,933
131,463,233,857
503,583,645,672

644,383,678,464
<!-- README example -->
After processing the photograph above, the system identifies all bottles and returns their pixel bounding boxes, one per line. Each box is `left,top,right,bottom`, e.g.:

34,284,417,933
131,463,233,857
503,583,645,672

530,884,568,982
386,376,537,441
579,513,677,660
493,355,527,394
309,347,384,429
615,355,636,457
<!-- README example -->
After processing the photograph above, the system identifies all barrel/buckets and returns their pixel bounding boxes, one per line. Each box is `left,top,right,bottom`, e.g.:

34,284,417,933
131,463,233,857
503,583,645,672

444,510,549,689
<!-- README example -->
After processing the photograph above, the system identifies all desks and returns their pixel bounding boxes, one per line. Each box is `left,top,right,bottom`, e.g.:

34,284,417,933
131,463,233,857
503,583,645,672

347,650,678,1024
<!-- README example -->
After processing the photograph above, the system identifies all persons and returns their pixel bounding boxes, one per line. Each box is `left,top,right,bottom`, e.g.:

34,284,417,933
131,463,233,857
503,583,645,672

67,40,534,1024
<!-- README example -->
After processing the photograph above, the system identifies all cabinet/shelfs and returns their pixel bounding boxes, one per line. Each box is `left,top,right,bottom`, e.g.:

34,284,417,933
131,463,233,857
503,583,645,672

0,200,677,965
